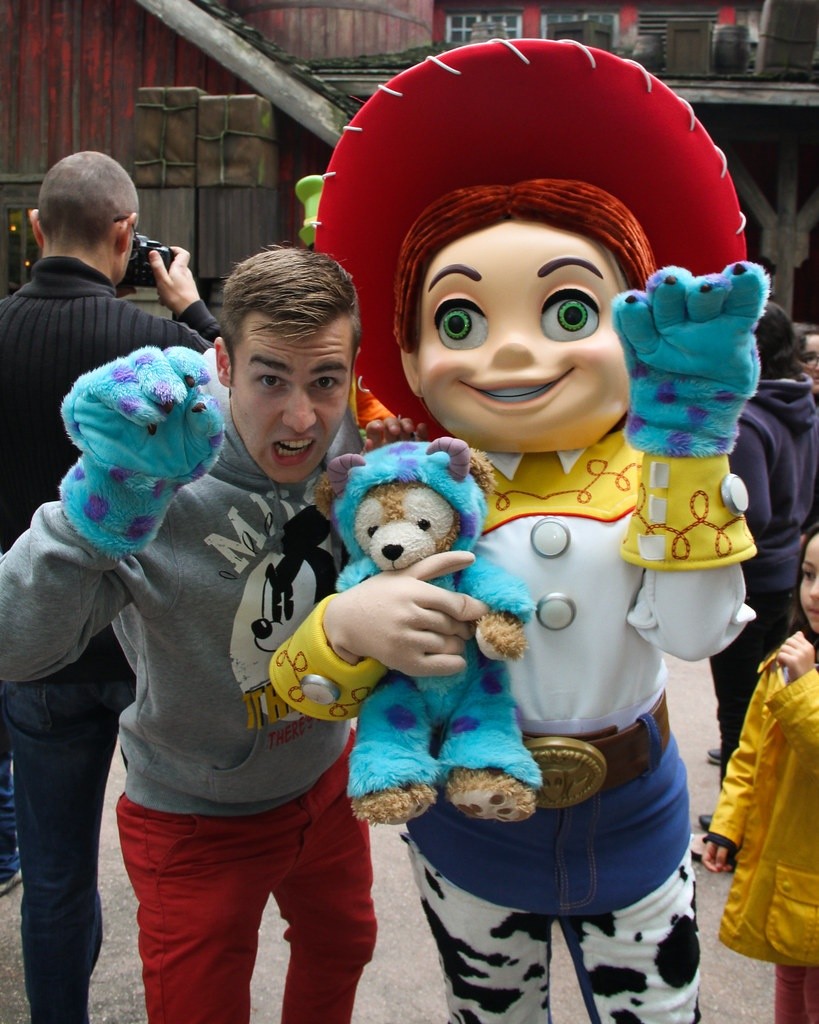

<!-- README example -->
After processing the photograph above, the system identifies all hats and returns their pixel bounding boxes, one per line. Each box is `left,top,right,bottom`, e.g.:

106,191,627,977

312,38,749,448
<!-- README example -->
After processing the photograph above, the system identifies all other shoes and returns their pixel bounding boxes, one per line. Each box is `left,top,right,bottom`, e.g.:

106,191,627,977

699,815,713,830
707,748,722,765
689,832,710,860
0,867,22,896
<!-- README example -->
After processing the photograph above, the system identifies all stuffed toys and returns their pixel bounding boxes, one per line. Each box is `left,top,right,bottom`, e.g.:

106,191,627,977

314,437,540,823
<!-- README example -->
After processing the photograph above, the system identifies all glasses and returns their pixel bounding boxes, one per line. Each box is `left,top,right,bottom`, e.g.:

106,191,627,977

800,355,819,366
114,215,141,260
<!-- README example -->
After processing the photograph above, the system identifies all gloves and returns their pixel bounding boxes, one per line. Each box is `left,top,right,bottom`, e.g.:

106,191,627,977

59,346,224,560
609,263,770,458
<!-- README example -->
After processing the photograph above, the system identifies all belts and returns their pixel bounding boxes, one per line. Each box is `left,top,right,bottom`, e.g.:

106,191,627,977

514,690,670,810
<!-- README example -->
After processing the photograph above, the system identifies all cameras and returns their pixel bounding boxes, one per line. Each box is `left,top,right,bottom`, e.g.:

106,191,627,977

114,235,174,287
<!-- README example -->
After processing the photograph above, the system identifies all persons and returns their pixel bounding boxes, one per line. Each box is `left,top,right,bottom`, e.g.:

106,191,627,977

269,40,769,1024
0,152,220,1024
689,303,819,1024
0,246,429,1024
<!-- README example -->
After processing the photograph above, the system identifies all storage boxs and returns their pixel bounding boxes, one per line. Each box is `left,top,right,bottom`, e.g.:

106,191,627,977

137,186,196,279
754,0,819,76
133,86,205,186
543,19,611,51
198,95,279,188
666,18,714,74
196,185,281,279
